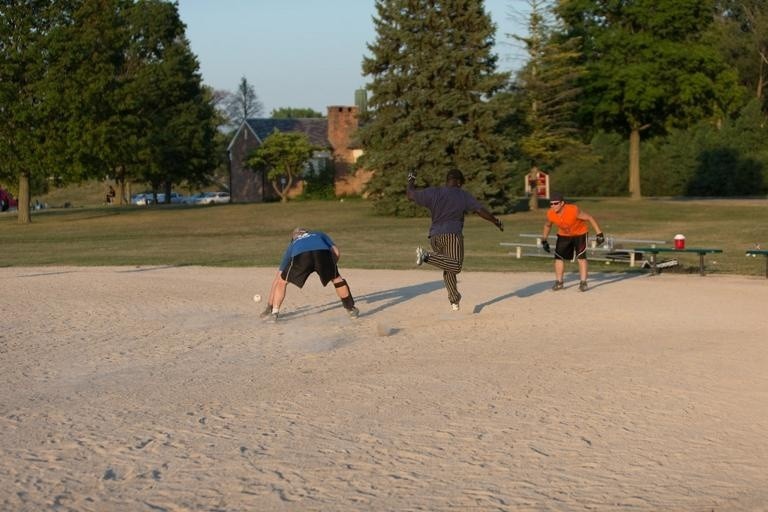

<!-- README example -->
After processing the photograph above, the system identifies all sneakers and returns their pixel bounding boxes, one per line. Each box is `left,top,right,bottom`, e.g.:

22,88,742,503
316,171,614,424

348,308,358,318
552,280,563,290
580,281,587,291
451,303,459,311
416,246,428,265
264,314,278,323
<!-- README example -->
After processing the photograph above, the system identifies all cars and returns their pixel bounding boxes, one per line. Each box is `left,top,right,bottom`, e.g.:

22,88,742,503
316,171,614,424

130,190,230,207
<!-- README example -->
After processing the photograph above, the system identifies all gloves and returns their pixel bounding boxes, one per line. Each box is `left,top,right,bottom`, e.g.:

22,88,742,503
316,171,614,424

408,173,416,183
595,233,603,245
542,240,551,253
495,219,504,232
262,305,272,315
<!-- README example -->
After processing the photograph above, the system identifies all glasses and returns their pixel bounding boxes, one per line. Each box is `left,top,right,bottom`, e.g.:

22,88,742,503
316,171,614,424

550,202,561,205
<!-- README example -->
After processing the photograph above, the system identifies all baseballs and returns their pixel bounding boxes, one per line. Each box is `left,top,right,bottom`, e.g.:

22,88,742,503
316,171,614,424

253,294,262,303
603,244,609,249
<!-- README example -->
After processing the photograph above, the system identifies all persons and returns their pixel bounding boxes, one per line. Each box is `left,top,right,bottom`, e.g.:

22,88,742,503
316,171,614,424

259,226,360,325
541,193,605,293
407,169,504,312
105,186,116,204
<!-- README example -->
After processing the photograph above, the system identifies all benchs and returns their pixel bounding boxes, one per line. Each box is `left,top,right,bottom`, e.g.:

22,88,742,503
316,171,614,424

634,246,768,277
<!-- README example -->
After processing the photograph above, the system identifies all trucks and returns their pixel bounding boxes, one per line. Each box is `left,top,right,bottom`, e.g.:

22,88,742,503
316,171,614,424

1,180,19,213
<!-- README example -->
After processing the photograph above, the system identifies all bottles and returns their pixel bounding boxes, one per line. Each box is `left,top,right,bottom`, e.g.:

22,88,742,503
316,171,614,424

674,233,685,251
